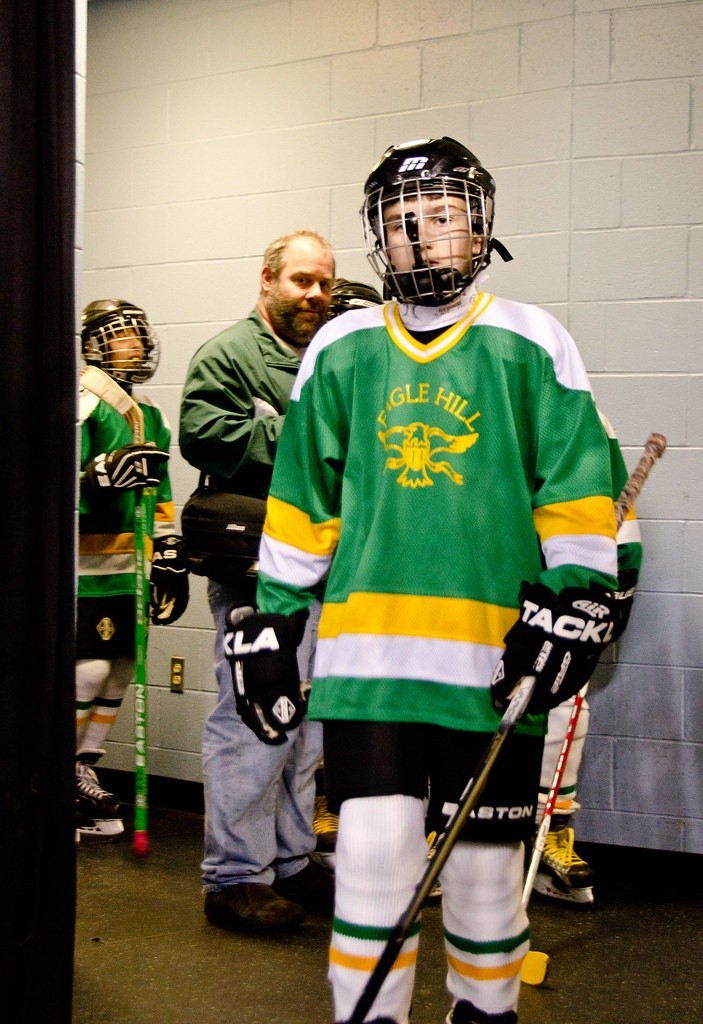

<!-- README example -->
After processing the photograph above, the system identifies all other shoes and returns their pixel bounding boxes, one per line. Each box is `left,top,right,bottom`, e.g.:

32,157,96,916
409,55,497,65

203,862,335,934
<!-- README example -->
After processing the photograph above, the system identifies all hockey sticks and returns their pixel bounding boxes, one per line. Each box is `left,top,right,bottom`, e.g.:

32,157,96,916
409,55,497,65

343,431,669,1024
79,362,153,858
521,679,591,985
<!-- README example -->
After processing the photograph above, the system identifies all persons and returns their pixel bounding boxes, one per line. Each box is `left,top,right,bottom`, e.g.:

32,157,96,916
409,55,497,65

72,300,188,841
427,411,643,903
225,137,617,1023
180,232,320,928
312,278,385,868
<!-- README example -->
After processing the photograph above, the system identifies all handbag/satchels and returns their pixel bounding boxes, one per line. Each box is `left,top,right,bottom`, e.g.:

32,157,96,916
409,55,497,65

181,484,268,565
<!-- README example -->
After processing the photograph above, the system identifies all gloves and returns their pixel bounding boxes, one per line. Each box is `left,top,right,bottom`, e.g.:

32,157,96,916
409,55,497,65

490,581,614,722
614,569,639,638
150,535,189,625
223,606,311,746
80,444,169,511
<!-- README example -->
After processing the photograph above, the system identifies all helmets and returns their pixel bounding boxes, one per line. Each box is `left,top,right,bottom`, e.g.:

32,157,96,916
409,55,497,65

328,279,383,321
359,136,513,308
81,300,160,384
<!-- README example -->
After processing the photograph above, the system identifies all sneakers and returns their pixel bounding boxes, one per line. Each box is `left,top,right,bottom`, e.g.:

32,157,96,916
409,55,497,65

313,795,339,853
76,748,118,804
527,812,591,887
425,832,444,866
